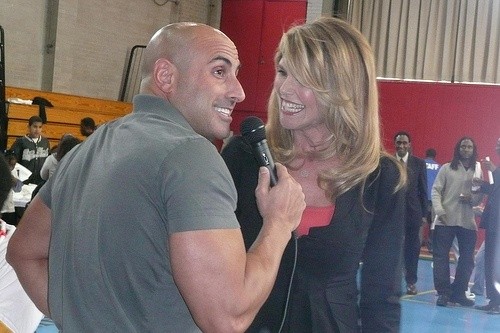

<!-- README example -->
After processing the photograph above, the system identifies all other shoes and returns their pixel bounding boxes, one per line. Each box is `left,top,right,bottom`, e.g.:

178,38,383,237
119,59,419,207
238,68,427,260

476,301,500,313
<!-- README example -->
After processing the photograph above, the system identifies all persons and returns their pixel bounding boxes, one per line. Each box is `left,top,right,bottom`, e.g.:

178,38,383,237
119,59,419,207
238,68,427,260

221,15,406,332
418,148,441,252
431,136,484,307
40,135,80,181
80,118,96,136
9,115,51,184
392,130,432,294
472,137,500,314
5,22,306,333
470,202,485,296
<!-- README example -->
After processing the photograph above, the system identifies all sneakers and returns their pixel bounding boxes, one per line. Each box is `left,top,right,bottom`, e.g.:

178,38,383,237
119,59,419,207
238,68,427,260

449,293,475,306
436,294,448,306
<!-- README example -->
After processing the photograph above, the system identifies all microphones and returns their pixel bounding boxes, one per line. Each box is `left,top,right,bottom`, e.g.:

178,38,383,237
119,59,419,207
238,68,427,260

239,115,298,240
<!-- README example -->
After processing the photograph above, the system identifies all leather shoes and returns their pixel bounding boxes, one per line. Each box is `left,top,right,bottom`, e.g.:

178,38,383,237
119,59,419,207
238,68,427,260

406,284,417,295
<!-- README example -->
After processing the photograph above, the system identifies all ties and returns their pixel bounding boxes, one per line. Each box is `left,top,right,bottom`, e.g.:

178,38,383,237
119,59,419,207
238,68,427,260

400,159,404,165
